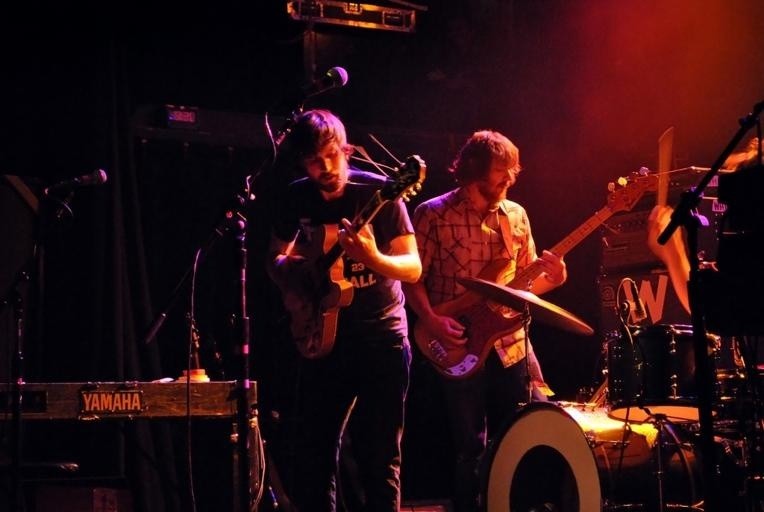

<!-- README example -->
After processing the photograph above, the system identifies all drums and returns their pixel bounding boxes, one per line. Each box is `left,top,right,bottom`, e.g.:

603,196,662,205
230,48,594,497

604,321,722,424
476,397,718,512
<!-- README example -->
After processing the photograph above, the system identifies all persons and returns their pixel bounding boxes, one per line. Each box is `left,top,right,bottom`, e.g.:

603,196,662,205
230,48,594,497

401,128,568,512
644,126,760,320
261,107,423,512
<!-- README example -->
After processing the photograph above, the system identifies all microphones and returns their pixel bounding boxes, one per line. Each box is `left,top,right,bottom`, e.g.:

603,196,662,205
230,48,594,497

73,168,108,186
655,413,683,448
623,280,648,323
298,66,350,104
541,500,559,512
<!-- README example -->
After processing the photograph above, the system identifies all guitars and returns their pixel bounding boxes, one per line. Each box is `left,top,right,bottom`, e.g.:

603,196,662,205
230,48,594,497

413,166,655,377
293,155,427,360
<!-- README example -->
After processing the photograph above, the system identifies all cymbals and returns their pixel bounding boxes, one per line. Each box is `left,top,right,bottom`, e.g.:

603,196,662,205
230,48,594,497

460,274,597,339
